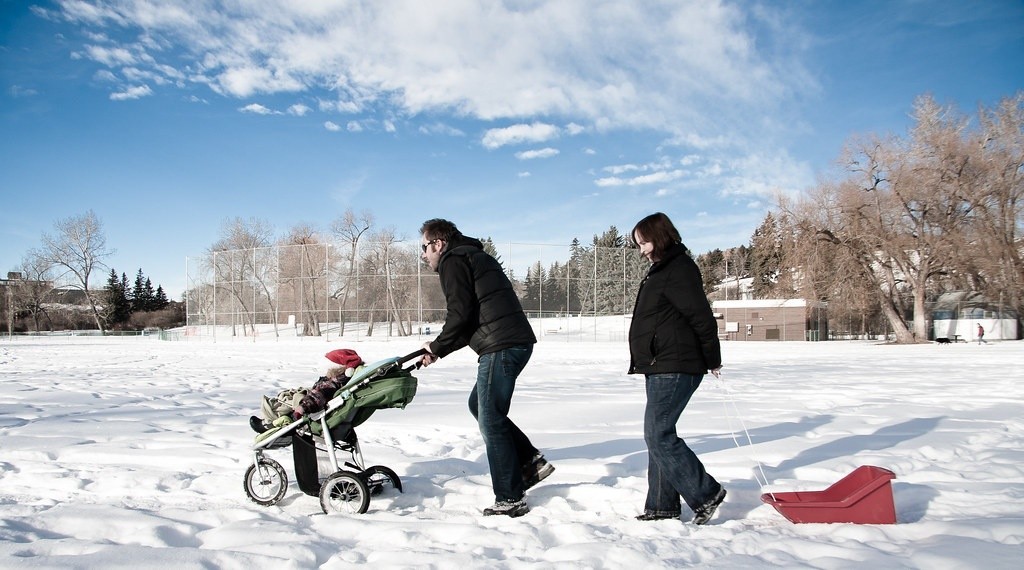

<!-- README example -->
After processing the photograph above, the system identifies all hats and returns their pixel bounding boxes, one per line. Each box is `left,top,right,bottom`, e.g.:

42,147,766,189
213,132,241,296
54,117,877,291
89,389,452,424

323,349,361,377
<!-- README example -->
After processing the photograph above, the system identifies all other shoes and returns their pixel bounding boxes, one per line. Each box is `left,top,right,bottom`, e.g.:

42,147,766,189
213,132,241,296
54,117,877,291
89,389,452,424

635,514,680,521
249,416,268,434
691,488,727,525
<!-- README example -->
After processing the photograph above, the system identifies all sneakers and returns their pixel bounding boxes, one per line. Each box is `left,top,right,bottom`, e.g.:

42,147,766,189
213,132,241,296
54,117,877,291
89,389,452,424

483,498,529,517
521,454,555,491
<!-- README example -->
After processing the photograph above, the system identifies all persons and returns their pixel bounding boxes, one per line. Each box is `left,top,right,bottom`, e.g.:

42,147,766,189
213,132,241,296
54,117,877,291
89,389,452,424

250,349,365,433
420,219,555,518
629,212,726,525
977,323,987,345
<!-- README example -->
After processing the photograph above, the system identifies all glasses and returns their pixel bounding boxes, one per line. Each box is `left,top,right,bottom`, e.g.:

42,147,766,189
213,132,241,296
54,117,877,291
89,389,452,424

422,239,435,253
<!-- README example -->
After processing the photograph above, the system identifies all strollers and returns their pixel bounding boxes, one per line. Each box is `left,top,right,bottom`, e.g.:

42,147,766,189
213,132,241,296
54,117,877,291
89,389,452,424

243,348,428,518
